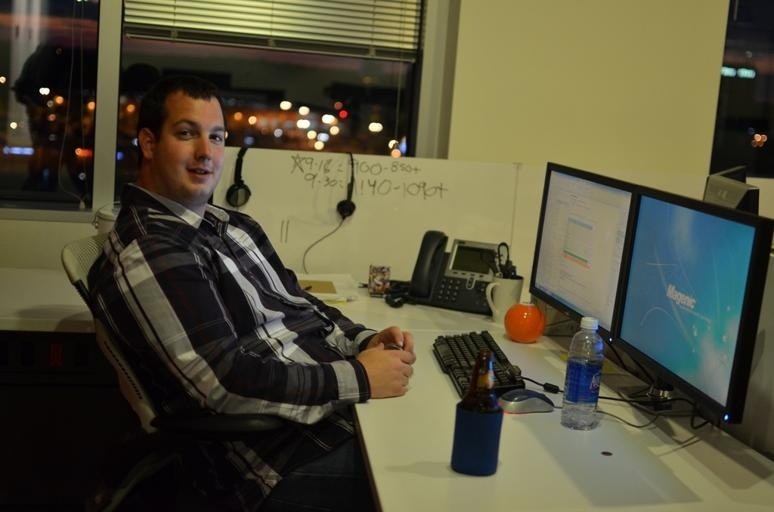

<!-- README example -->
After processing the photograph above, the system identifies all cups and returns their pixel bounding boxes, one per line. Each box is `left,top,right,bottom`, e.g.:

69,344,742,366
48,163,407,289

485,276,524,325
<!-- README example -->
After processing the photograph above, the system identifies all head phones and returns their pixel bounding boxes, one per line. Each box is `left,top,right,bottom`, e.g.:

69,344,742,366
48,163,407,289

337,154,357,219
226,144,252,208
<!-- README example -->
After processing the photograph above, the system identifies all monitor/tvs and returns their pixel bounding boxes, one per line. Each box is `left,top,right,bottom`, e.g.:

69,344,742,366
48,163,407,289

609,184,774,425
702,174,760,216
702,165,747,200
528,161,637,345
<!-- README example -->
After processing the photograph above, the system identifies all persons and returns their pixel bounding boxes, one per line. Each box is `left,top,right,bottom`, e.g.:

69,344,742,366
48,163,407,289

10,39,74,189
84,68,416,511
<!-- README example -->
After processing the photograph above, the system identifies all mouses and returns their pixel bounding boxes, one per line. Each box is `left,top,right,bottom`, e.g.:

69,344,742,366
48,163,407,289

497,389,555,415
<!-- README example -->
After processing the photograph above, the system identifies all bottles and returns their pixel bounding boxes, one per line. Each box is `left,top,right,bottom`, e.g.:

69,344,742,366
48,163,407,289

565,316,605,430
449,347,504,477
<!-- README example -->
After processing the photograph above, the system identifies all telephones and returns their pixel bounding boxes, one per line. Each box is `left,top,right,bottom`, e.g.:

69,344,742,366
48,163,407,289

402,229,509,316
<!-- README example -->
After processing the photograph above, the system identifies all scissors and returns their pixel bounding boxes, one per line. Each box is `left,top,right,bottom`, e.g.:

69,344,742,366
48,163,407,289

480,243,509,280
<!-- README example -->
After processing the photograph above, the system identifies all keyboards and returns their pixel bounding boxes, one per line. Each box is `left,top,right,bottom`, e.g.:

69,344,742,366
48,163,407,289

431,330,526,400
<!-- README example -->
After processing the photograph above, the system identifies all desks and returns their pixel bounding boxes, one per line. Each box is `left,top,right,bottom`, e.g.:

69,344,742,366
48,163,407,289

0,144,773,511
